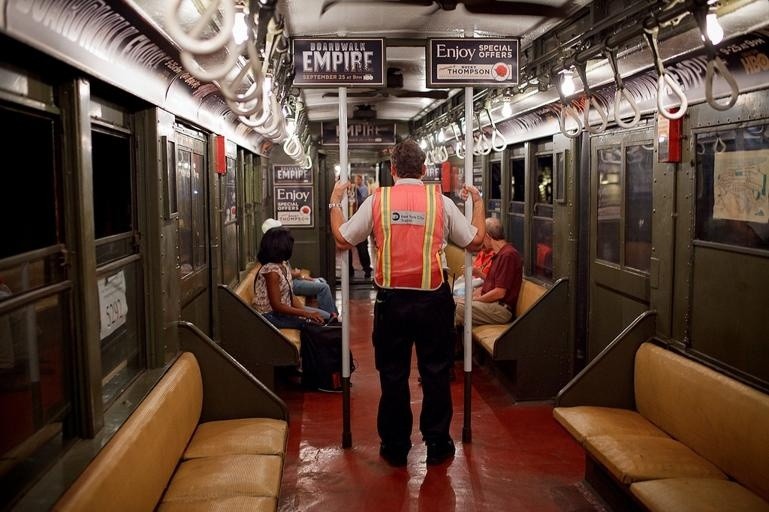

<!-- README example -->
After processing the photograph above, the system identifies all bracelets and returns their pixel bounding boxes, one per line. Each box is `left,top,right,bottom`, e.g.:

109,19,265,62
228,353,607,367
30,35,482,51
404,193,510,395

329,203,343,209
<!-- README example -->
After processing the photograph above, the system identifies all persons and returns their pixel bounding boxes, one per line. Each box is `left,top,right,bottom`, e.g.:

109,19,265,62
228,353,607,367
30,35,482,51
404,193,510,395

451,245,496,297
329,139,486,466
418,217,522,382
262,218,339,318
338,174,373,272
252,227,352,393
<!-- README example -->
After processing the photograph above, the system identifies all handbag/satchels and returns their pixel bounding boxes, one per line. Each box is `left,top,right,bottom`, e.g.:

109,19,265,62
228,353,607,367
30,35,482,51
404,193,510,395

297,315,325,327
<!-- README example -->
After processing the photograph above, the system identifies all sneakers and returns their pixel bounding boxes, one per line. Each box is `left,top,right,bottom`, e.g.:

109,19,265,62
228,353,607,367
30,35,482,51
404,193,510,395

380,439,407,465
318,382,344,393
427,435,455,464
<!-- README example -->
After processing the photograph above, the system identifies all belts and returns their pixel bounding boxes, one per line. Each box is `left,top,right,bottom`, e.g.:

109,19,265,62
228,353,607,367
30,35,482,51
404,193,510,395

498,301,513,314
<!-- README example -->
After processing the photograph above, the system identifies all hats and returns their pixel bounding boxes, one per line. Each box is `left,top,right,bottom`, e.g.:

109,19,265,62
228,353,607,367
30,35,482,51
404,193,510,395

262,218,282,234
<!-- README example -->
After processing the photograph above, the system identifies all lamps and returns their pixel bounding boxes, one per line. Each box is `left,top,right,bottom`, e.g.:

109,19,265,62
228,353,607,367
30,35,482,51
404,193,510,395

561,68,576,97
700,9,724,46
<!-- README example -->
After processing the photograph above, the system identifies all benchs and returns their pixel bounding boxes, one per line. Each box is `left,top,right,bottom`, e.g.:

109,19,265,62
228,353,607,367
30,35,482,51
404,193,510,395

50,321,291,512
445,240,569,402
552,310,769,511
217,263,311,401
